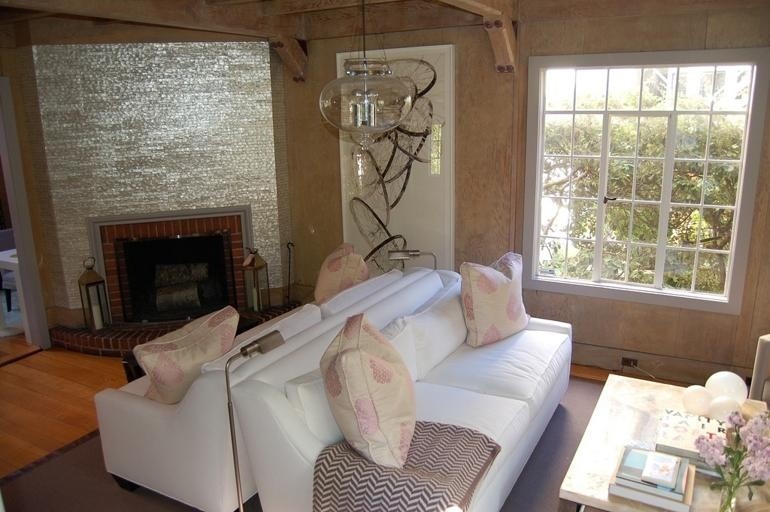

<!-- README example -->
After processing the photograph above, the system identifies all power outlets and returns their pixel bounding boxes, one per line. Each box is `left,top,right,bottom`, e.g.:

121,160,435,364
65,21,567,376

622,357,637,368
318,1,412,151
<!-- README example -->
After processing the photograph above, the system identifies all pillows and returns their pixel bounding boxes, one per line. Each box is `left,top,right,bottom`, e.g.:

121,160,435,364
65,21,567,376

322,267,403,317
320,314,417,470
457,252,530,348
286,316,418,447
131,306,241,404
200,304,322,373
407,278,469,381
313,243,370,305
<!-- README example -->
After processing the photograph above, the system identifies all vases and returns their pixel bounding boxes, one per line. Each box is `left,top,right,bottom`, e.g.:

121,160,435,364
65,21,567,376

718,485,737,512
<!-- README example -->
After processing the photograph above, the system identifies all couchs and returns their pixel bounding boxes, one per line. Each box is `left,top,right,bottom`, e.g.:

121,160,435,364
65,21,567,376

94,262,434,512
230,270,573,512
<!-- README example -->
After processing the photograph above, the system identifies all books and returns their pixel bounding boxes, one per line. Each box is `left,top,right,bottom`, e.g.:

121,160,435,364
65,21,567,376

608,408,731,511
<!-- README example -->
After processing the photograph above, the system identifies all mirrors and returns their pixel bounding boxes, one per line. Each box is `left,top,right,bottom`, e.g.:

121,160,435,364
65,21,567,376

0,0,284,511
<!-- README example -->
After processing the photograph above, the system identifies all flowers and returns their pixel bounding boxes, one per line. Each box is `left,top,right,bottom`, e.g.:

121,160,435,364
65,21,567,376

694,409,770,512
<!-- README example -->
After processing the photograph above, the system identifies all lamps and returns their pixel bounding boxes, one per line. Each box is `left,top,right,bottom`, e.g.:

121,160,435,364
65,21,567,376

76,256,113,334
240,246,272,312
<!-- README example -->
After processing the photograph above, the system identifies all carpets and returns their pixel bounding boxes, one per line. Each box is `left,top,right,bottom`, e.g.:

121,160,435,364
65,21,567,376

0,427,202,512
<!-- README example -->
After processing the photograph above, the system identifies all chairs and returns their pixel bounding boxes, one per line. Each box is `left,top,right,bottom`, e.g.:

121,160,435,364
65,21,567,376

748,333,770,410
2,271,17,312
